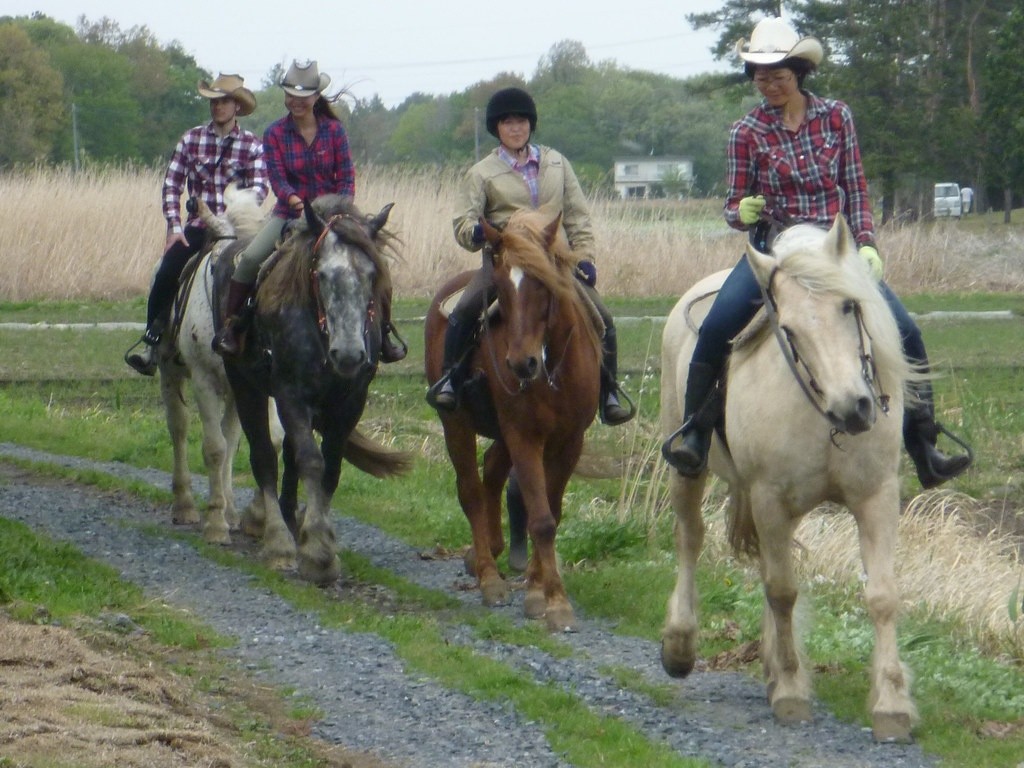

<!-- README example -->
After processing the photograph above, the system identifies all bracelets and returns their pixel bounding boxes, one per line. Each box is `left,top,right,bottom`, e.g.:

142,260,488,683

292,200,304,212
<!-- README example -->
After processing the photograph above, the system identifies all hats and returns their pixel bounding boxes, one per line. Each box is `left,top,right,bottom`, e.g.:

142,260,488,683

274,59,332,97
196,72,259,120
734,15,824,70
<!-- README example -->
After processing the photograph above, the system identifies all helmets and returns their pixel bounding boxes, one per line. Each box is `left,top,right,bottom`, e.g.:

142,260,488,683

486,88,537,141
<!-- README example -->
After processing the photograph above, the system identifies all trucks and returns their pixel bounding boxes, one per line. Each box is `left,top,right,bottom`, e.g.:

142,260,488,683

933,182,963,220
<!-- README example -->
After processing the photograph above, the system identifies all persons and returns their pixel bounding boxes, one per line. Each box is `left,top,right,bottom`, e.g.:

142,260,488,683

131,71,270,369
435,88,630,420
961,183,973,217
220,60,406,362
672,15,969,491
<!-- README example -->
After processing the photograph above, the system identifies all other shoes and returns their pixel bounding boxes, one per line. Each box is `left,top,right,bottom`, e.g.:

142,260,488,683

128,349,156,376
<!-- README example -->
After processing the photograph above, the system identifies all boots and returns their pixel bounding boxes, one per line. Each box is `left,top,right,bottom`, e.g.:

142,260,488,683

670,362,720,479
377,288,409,361
599,327,630,422
432,313,473,409
217,276,253,355
901,376,969,489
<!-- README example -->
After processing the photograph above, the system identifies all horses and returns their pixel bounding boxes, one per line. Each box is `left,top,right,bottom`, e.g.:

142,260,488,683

657,211,945,744
213,195,410,585
154,183,286,547
425,207,605,634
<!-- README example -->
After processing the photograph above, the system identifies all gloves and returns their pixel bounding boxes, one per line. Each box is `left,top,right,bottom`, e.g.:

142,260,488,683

471,221,494,243
575,260,598,287
740,194,767,226
859,245,883,285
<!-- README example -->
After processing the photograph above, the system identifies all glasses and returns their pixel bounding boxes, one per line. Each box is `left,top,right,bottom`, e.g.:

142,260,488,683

752,74,794,86
